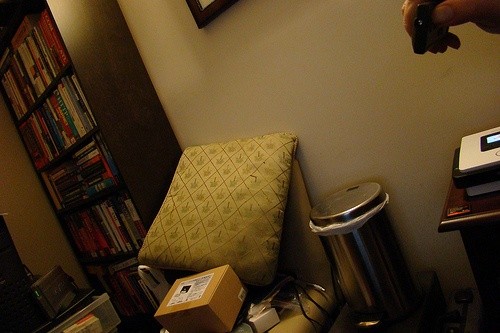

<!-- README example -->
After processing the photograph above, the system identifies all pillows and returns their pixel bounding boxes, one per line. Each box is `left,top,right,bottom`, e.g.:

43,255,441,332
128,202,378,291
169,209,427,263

137,131,298,286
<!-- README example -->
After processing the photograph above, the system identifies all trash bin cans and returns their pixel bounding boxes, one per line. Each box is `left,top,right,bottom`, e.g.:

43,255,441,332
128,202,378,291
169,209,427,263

309,182,421,333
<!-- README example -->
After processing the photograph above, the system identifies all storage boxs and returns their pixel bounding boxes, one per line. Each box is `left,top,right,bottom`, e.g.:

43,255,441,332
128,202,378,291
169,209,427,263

154,265,249,333
249,308,280,333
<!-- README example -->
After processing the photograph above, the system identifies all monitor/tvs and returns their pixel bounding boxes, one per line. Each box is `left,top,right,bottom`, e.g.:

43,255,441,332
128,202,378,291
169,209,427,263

0,215,57,333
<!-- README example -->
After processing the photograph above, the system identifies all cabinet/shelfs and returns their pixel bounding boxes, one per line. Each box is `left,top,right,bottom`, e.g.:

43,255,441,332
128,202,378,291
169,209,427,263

0,0,184,333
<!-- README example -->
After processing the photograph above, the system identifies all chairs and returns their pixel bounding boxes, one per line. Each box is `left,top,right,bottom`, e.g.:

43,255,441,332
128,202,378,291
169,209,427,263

159,159,337,333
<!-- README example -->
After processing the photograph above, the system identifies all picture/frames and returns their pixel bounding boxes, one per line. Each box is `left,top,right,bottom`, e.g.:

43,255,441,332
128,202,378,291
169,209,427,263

185,0,239,29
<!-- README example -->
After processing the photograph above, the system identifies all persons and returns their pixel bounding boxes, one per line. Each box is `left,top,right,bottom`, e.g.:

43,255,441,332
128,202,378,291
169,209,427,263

400,0,500,54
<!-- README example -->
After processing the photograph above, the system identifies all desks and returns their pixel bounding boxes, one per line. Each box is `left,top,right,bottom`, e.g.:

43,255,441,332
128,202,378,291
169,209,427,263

438,179,500,333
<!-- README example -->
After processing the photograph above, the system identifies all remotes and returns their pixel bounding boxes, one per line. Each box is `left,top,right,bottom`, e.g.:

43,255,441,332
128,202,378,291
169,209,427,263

412,2,448,54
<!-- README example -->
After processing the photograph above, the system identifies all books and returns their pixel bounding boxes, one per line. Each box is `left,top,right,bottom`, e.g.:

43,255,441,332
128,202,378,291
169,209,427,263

0,10,173,322
